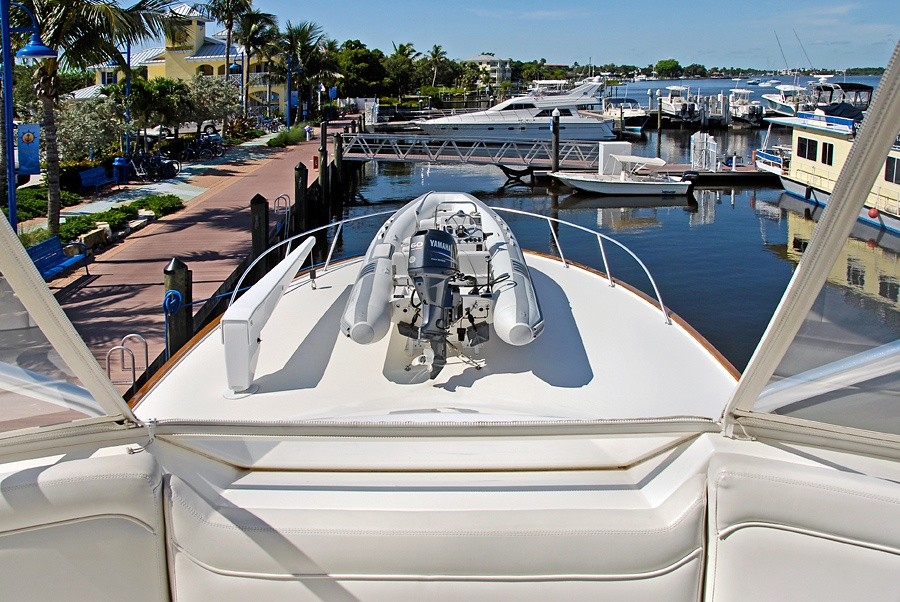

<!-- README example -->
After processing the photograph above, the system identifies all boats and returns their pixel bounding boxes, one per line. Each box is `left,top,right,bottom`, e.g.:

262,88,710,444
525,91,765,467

544,153,692,197
629,131,770,188
751,102,899,231
412,65,876,134
0,38,900,600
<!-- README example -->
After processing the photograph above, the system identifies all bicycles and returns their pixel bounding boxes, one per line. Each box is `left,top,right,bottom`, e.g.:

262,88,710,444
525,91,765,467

126,114,284,183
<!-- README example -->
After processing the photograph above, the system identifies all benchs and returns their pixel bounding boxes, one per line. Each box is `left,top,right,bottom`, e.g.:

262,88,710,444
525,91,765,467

26,233,91,285
78,166,117,198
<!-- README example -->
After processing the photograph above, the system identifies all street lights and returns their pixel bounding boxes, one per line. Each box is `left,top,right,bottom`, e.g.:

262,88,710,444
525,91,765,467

0,0,56,243
229,50,244,120
285,55,303,134
